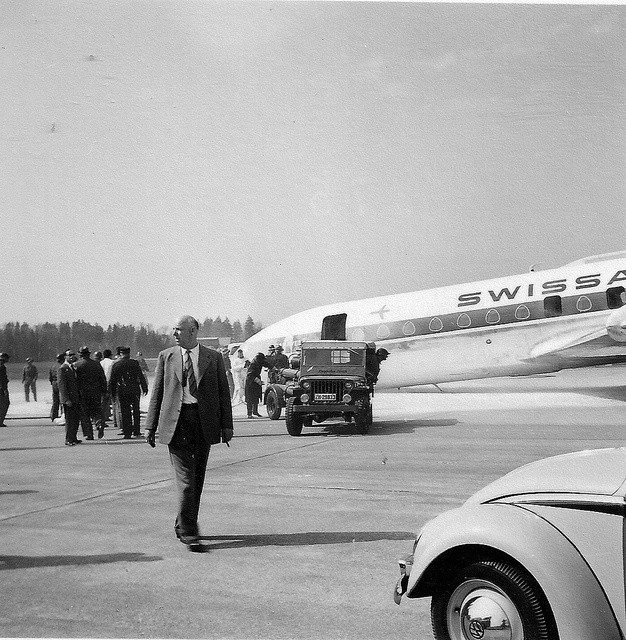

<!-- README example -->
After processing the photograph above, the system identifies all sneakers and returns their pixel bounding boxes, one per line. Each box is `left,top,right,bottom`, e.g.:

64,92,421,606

104,425,109,427
253,412,262,417
117,433,125,435
124,434,131,438
248,416,255,419
114,424,116,427
85,435,94,440
98,425,104,438
134,433,141,435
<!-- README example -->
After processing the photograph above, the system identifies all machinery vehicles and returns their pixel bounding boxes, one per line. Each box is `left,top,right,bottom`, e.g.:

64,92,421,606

264,340,388,435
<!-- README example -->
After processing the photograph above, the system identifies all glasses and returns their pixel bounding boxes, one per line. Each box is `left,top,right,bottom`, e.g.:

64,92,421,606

64,354,75,357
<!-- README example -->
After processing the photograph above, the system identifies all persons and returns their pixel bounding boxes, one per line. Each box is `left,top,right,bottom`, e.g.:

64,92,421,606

99,349,115,421
244,353,267,419
21,356,39,403
106,347,129,435
134,350,150,375
92,351,102,363
221,348,235,400
109,346,148,439
72,346,107,440
231,349,250,405
266,343,288,369
368,348,391,382
144,313,233,551
48,353,65,422
56,349,82,446
0,352,11,427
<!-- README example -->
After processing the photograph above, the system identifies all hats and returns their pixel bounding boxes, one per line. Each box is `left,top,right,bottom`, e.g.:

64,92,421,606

0,353,10,358
135,351,143,357
275,345,284,350
222,348,230,355
377,348,390,356
56,353,64,359
268,345,275,351
77,345,92,354
94,352,102,358
120,346,130,353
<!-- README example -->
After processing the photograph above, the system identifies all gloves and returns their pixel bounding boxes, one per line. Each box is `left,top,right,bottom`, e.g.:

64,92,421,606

254,377,264,387
271,366,279,373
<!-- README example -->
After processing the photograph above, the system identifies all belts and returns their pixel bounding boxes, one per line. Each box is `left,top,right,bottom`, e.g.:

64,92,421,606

182,403,199,407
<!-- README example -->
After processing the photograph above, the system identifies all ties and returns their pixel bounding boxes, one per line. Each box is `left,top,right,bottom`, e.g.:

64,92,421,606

71,364,76,372
182,350,198,398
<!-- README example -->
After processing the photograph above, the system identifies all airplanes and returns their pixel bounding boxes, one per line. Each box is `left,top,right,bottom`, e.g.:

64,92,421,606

232,250,625,388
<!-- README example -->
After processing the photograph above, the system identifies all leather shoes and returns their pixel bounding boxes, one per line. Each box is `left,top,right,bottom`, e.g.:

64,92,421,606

71,440,82,444
177,532,186,544
65,441,74,445
188,540,202,552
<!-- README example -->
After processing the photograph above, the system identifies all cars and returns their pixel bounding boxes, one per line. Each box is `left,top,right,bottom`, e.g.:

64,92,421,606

395,447,625,640
